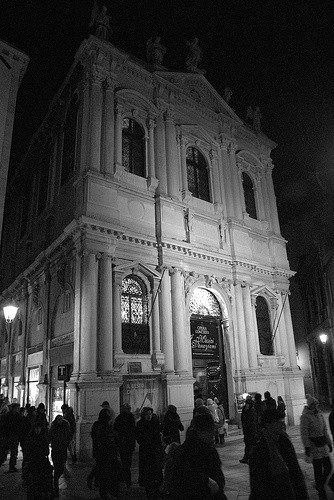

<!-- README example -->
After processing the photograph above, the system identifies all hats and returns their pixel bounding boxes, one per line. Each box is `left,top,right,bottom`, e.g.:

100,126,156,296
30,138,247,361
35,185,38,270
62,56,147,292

36,403,46,412
189,413,219,429
140,407,153,417
100,401,109,407
305,393,318,406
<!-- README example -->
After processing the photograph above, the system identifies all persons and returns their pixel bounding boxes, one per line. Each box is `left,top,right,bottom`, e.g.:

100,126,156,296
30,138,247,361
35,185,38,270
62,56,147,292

87,3,262,132
245,430,310,500
300,393,333,500
163,413,229,500
0,394,185,500
193,391,334,465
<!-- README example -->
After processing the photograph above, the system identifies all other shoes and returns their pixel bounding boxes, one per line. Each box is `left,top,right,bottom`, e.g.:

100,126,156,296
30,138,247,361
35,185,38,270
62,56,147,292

239,460,248,464
313,482,320,490
319,493,328,500
87,475,92,489
71,454,77,464
9,466,18,471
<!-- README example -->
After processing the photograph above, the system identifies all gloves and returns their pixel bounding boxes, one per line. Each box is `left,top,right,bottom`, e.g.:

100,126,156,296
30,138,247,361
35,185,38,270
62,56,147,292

305,447,312,455
328,443,333,452
